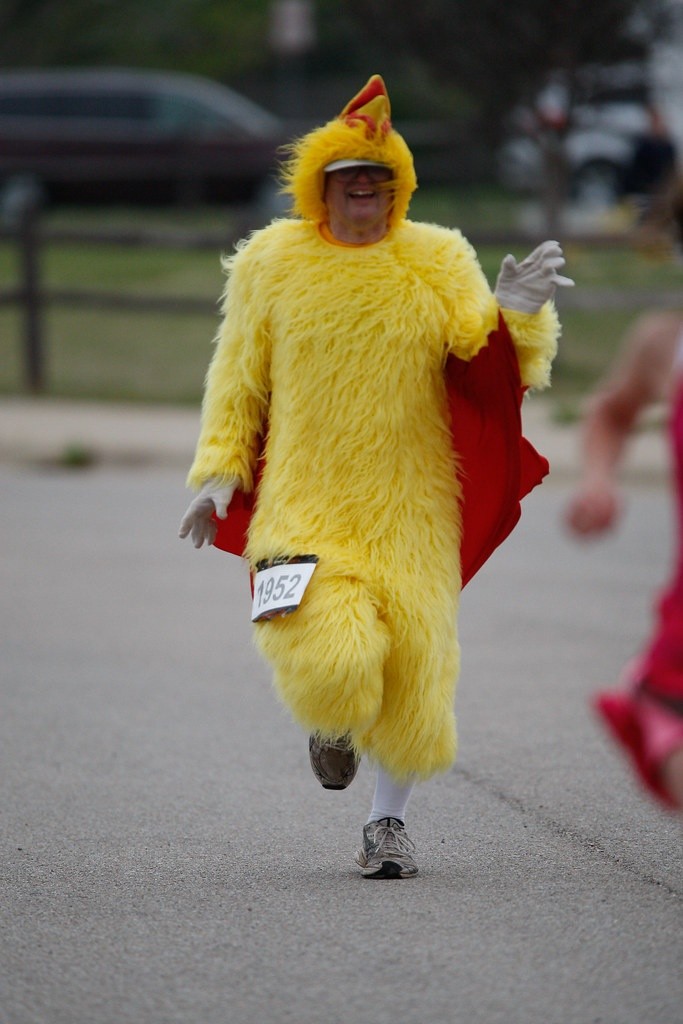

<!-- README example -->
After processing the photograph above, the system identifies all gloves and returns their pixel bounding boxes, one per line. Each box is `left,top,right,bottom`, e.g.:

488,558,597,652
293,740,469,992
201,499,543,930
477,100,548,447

179,472,238,549
490,240,576,314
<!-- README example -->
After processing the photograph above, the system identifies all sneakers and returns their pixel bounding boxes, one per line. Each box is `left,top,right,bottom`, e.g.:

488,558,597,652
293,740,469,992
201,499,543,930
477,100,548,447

308,724,360,791
355,815,418,881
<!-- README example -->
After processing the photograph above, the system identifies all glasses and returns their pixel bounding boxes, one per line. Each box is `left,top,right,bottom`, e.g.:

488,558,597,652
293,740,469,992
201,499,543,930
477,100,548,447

331,170,387,188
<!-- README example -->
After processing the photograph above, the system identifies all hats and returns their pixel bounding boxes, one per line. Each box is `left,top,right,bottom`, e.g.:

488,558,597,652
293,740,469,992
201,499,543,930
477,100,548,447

323,157,390,174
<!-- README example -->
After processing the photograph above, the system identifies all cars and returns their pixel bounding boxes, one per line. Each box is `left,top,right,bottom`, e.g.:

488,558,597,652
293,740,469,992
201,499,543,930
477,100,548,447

0,66,307,210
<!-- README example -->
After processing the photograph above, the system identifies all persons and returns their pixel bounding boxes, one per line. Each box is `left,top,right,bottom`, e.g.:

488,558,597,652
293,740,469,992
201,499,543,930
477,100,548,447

178,75,575,880
570,177,683,815
